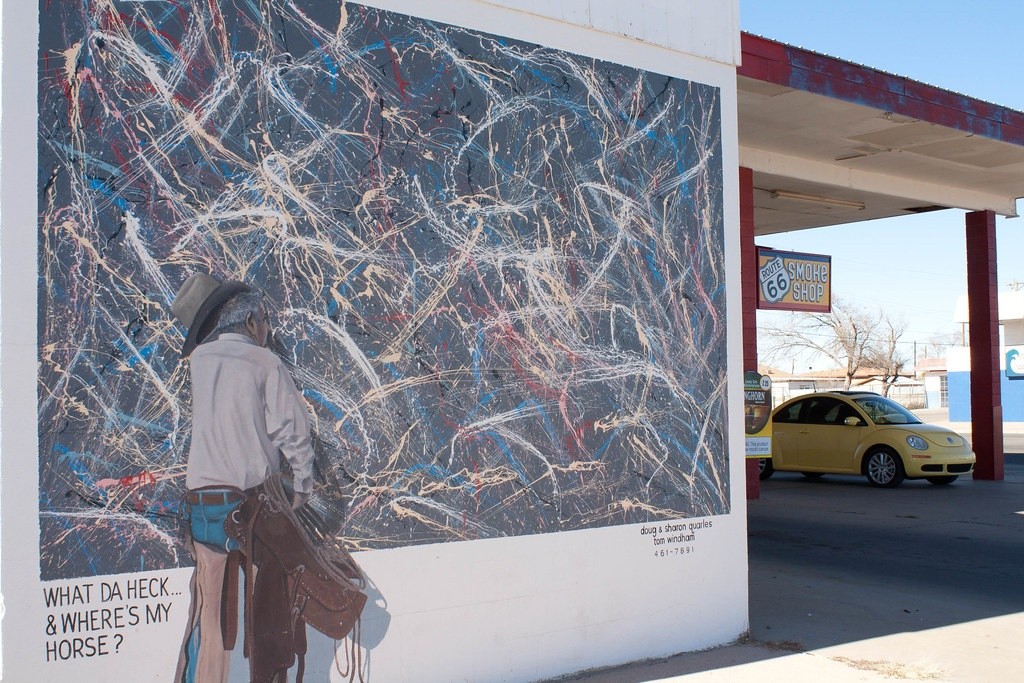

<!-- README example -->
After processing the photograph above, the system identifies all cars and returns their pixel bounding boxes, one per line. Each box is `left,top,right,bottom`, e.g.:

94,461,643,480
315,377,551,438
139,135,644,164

757,390,976,489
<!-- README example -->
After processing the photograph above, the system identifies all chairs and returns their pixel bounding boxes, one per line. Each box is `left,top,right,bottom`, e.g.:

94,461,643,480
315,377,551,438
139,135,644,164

836,404,854,426
